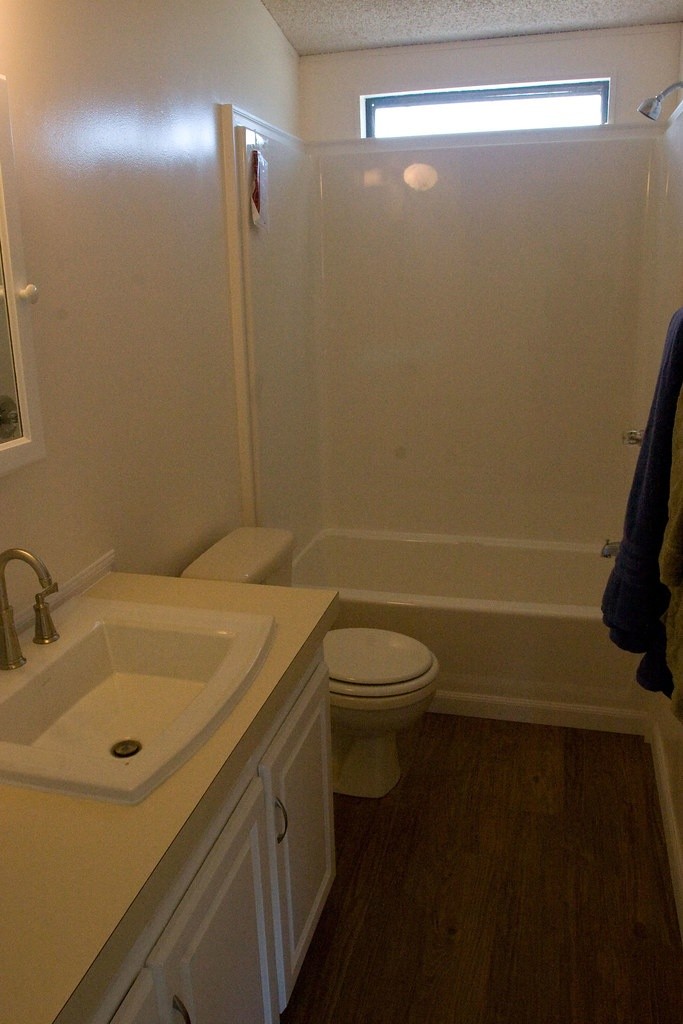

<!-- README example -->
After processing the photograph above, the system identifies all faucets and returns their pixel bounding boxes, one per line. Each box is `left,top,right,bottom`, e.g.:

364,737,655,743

601,541,623,559
0,549,53,670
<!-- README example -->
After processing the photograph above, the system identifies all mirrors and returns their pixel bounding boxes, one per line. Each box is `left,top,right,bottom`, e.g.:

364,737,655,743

0,72,50,481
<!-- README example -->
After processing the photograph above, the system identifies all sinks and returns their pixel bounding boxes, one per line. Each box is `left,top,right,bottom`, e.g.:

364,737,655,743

0,594,277,804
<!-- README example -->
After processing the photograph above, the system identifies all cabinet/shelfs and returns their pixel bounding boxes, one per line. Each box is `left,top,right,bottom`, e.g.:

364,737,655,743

101,637,338,1024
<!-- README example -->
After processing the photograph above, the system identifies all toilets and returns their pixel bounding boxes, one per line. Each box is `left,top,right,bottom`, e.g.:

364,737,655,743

179,525,441,799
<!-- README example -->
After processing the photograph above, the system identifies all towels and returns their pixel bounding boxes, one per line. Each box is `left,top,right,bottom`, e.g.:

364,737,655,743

600,309,683,720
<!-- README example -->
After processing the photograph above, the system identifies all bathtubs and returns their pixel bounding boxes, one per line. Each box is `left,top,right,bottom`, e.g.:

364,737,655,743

292,529,654,736
